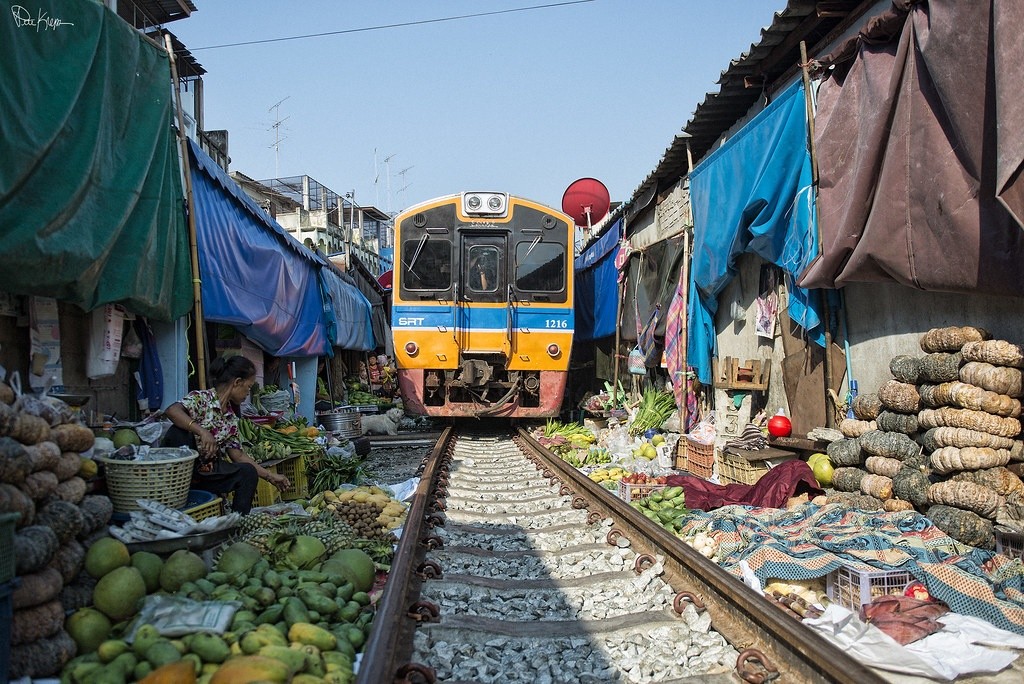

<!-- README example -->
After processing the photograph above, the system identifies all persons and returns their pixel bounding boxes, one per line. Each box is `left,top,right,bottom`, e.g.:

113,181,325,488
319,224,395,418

316,359,344,411
365,356,397,400
154,354,291,518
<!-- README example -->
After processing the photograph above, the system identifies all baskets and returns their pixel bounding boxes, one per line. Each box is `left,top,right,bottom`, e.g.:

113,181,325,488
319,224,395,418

0,512,21,584
92,446,202,515
0,577,14,684
242,410,285,424
617,479,667,504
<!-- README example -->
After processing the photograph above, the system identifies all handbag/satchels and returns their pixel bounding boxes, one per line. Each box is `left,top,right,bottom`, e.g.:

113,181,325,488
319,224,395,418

729,272,746,325
37,376,82,424
120,319,144,359
9,371,54,426
627,344,647,375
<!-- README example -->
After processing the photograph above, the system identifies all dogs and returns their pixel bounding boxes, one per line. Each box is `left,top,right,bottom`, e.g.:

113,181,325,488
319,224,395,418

359,408,404,436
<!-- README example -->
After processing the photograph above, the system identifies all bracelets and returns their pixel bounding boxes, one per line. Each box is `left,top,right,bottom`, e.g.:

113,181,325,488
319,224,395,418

187,420,196,432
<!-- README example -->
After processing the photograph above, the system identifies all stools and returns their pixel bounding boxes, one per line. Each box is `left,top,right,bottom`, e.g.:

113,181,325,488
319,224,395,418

712,356,771,390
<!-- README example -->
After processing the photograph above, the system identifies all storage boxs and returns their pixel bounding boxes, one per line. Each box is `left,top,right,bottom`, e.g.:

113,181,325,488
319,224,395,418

584,418,608,430
676,432,688,471
182,498,223,523
826,563,916,614
617,478,667,502
338,404,379,416
687,436,714,479
717,448,800,487
245,410,284,429
226,465,282,508
277,455,310,500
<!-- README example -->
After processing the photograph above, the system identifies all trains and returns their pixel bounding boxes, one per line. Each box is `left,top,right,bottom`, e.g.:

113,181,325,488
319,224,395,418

390,190,575,418
